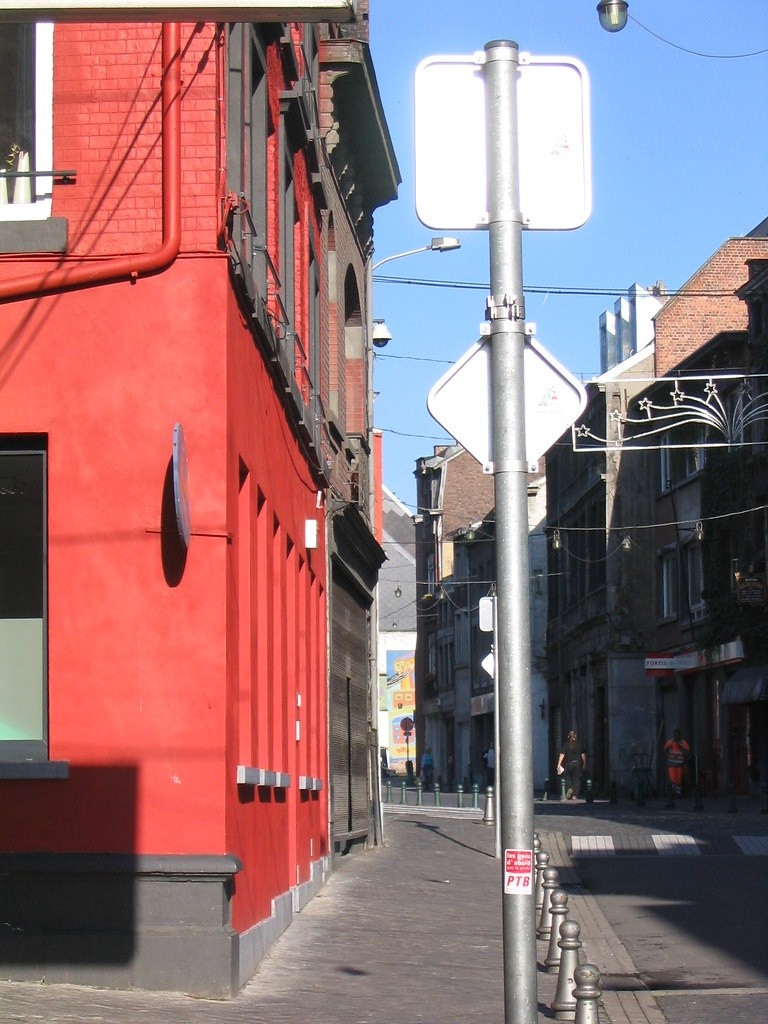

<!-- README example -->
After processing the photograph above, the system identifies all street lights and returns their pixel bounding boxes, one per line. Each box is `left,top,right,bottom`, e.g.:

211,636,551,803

364,237,461,849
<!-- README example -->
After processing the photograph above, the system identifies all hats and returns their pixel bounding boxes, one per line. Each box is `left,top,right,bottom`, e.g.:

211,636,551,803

424,745,432,751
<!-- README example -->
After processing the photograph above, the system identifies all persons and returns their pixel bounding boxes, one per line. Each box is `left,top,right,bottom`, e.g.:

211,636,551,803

481,741,495,786
421,748,435,792
664,730,689,803
557,731,587,800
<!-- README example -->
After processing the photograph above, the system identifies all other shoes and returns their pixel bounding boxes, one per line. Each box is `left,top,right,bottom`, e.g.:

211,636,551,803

566,789,573,798
572,795,576,800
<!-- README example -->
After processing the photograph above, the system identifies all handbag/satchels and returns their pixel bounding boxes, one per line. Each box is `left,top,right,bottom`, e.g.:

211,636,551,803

418,769,425,782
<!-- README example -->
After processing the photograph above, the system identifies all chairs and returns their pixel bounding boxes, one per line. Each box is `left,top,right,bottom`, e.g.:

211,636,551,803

633,754,656,796
